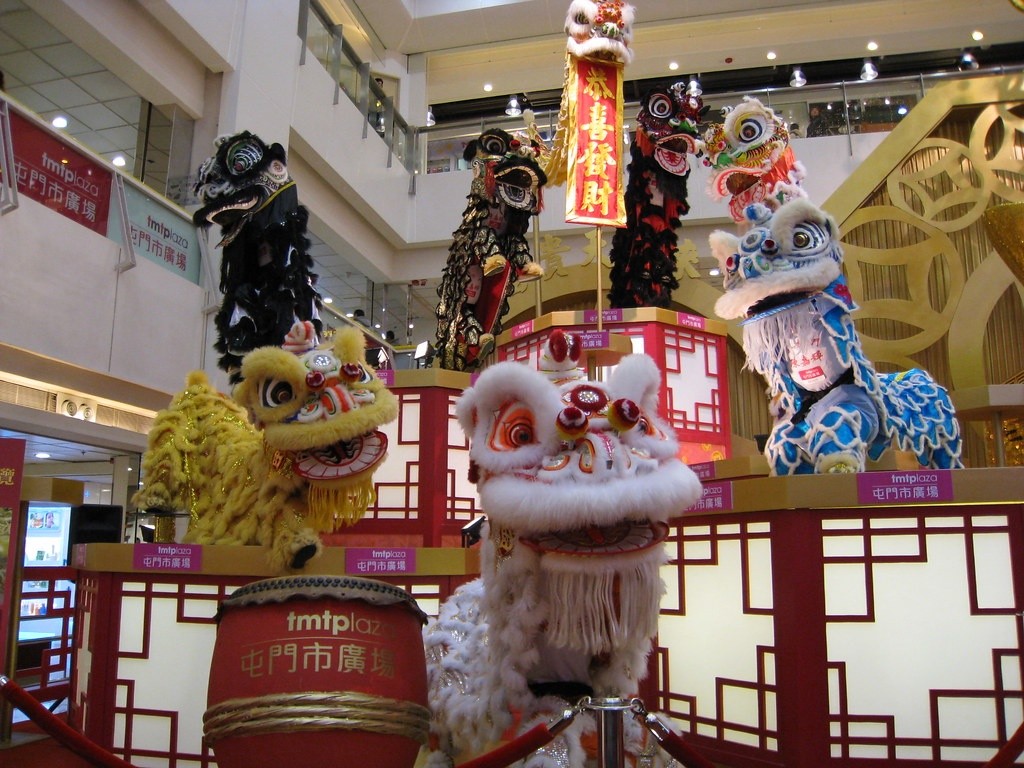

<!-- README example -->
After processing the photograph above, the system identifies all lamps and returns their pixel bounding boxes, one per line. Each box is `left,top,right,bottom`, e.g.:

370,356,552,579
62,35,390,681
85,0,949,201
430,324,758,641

139,524,155,542
365,347,390,369
686,74,702,98
506,94,521,116
426,106,435,127
462,514,486,548
789,64,807,88
414,340,436,370
376,116,385,133
860,57,878,81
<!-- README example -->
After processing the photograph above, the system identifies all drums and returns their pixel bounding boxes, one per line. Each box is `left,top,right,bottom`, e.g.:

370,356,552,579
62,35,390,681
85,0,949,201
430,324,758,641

201,573,431,768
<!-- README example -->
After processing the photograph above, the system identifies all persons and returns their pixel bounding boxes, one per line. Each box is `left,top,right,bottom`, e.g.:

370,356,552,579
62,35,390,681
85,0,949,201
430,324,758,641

354,309,373,329
368,77,384,131
790,123,803,139
384,330,401,347
807,106,830,137
